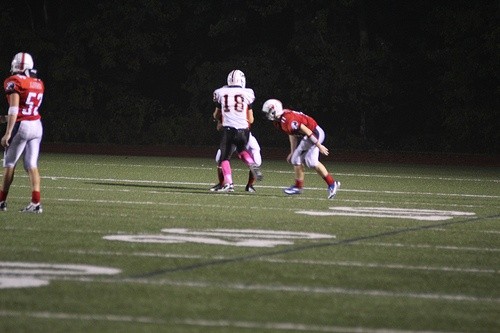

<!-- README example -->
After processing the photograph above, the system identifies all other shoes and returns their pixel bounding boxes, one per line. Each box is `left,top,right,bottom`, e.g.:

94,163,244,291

254,168,264,180
245,185,256,192
210,183,222,191
218,184,234,192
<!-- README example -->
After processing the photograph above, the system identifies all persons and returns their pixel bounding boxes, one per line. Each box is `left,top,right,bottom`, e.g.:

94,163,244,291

210,69,262,193
0,52,44,213
262,98,341,199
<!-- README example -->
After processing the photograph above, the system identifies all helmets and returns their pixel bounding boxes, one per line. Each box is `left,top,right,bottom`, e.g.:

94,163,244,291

261,99,283,121
227,70,246,88
10,52,34,73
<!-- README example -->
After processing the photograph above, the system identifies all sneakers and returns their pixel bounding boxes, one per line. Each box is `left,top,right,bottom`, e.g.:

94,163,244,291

18,201,42,214
284,186,303,195
328,181,341,198
0,200,7,212
